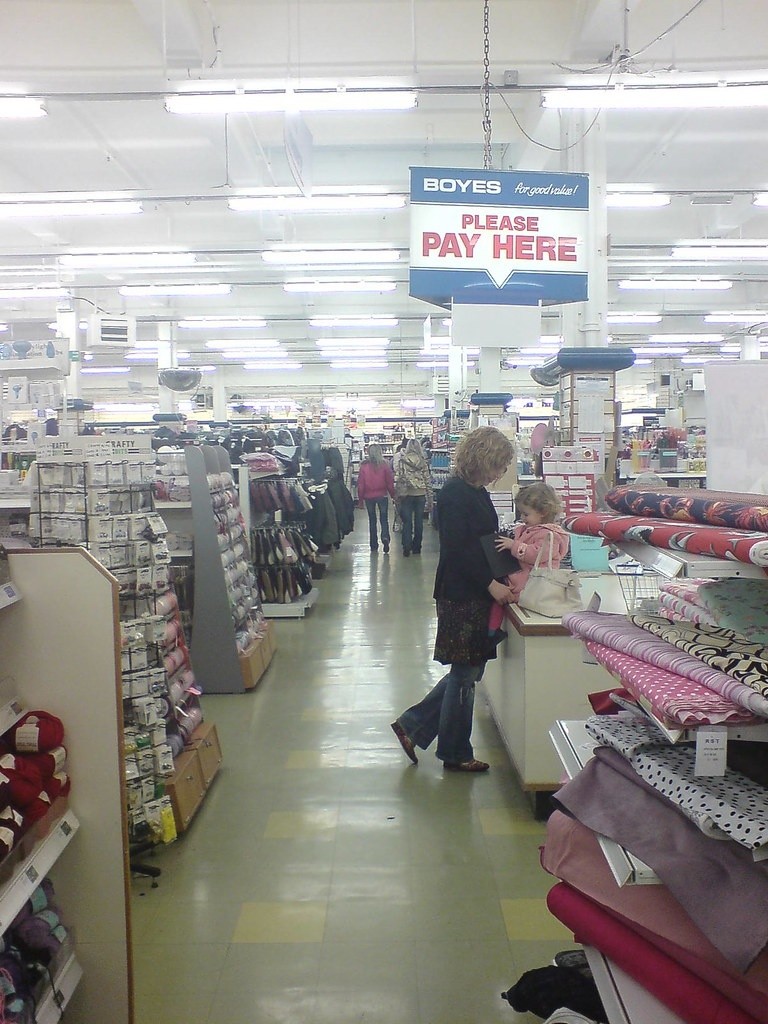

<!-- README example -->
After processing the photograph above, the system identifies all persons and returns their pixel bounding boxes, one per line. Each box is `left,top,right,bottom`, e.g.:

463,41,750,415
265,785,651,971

357,443,394,555
389,425,516,773
389,436,433,557
482,482,569,655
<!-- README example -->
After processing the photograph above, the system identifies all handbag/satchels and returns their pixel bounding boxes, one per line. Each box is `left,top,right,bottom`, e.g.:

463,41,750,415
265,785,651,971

395,475,409,500
518,531,586,618
393,509,404,534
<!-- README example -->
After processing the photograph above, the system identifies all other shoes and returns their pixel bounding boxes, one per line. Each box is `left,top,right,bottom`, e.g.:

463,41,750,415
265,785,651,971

383,540,390,553
442,759,492,771
391,719,420,763
371,543,380,551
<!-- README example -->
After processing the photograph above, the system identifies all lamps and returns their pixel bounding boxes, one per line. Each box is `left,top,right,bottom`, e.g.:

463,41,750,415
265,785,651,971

228,195,411,211
0,199,146,218
751,192,768,206
538,85,768,109
164,90,420,114
607,194,672,208
0,96,49,119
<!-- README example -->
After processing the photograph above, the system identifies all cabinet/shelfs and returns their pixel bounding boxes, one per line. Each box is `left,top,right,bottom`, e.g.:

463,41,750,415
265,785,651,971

0,358,768,1024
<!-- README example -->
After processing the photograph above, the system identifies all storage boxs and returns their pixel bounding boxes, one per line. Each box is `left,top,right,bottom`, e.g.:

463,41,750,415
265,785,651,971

253,630,272,671
28,423,46,449
49,380,65,406
260,621,278,656
185,721,223,790
7,375,29,404
240,642,264,688
28,383,49,408
165,750,207,832
